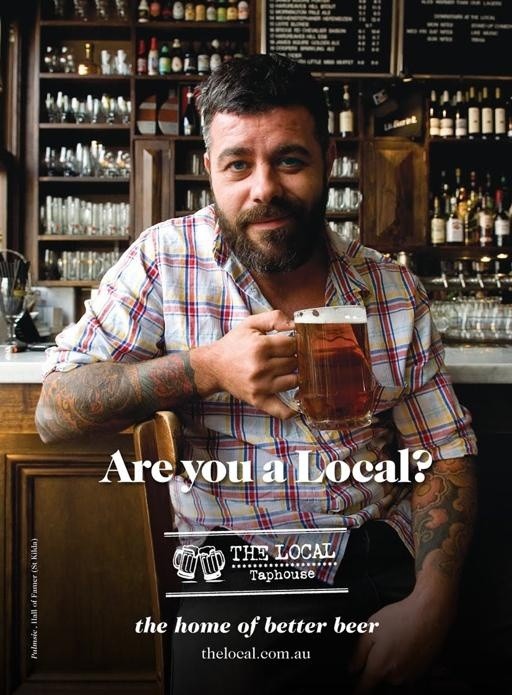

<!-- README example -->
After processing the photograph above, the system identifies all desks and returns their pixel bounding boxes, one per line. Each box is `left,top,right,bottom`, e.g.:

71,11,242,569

1,339,511,694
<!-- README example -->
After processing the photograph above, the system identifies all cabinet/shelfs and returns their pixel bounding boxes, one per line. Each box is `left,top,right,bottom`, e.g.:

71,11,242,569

25,20,512,334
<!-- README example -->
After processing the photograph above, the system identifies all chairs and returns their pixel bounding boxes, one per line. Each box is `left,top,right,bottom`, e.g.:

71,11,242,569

132,412,181,694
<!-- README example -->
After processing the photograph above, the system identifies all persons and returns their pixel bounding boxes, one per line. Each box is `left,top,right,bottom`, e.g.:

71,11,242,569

34,49,482,695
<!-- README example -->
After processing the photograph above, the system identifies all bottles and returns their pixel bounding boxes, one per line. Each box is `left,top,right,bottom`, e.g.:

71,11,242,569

499,175,507,188
173,0,185,22
183,86,198,137
44,44,78,76
206,0,216,22
161,1,173,22
469,170,476,187
339,84,355,139
78,41,100,75
494,188,512,248
39,195,130,237
184,46,198,75
148,35,159,76
172,38,184,75
430,195,445,248
441,169,447,184
455,87,467,142
159,44,172,75
477,191,494,247
455,166,461,186
494,87,505,140
137,38,147,75
442,183,449,203
507,91,512,140
197,52,210,75
150,1,161,22
440,88,455,141
481,85,494,141
470,189,476,205
429,89,440,141
39,140,130,180
101,47,132,74
137,88,161,136
237,2,249,23
185,0,195,22
446,195,463,247
211,52,222,73
224,53,233,67
464,199,477,246
196,0,207,22
216,0,227,22
41,91,133,127
467,85,481,142
158,87,180,137
455,187,460,201
227,1,237,22
138,0,151,23
322,86,335,138
457,185,467,220
482,173,493,209
43,248,125,284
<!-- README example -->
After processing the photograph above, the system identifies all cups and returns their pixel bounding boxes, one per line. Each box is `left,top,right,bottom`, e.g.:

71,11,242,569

42,249,123,281
45,139,130,178
41,195,129,236
0,277,29,353
270,303,378,432
44,90,133,123
428,297,512,343
